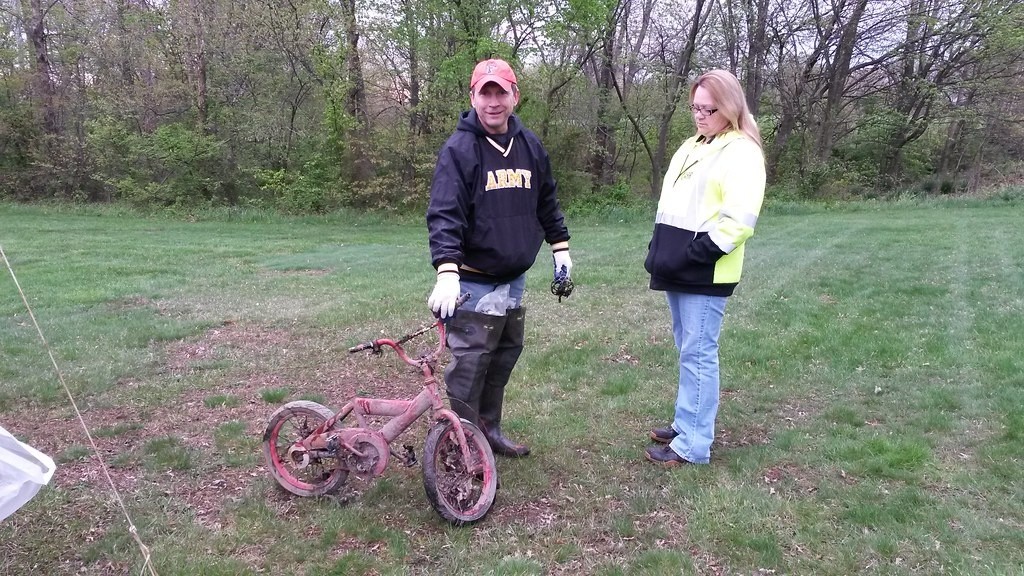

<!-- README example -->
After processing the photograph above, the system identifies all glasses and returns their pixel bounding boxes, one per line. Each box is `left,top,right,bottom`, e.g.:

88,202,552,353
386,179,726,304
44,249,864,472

689,104,718,116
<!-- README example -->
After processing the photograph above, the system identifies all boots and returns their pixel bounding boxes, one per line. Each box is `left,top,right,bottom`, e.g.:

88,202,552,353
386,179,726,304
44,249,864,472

440,305,507,489
478,306,530,458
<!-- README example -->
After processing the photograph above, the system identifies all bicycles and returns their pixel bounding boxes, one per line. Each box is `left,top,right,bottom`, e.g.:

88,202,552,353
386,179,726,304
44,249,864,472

260,291,498,525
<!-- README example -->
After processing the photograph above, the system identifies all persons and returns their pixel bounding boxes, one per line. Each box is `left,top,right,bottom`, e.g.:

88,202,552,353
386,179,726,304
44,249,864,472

643,70,767,465
426,59,573,489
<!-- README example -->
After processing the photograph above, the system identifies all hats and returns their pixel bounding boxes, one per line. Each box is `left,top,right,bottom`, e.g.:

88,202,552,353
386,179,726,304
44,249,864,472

470,59,516,93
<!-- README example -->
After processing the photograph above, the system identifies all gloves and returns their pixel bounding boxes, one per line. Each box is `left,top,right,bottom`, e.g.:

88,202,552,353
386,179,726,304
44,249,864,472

427,270,460,318
553,248,573,278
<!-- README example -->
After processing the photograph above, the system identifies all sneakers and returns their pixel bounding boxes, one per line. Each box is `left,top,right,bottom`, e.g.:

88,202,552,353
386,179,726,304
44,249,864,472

650,426,678,443
644,444,695,465
